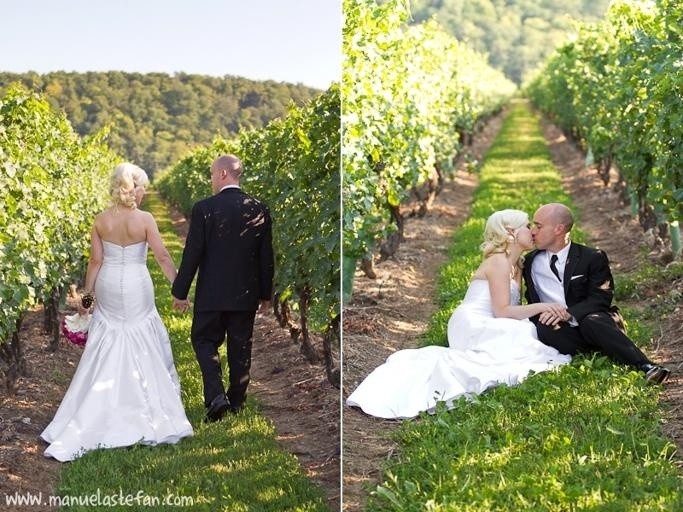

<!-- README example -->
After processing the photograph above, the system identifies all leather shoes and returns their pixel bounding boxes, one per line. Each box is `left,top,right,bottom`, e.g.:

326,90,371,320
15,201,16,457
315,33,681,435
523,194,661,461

642,363,670,384
205,393,230,422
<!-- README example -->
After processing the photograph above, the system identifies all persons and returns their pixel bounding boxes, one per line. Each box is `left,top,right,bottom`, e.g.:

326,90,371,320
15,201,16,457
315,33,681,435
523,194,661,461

40,161,191,463
172,154,274,426
447,209,568,386
523,203,670,386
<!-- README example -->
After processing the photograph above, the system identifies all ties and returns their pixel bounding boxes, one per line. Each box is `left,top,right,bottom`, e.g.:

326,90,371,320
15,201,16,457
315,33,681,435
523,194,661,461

550,255,561,282
